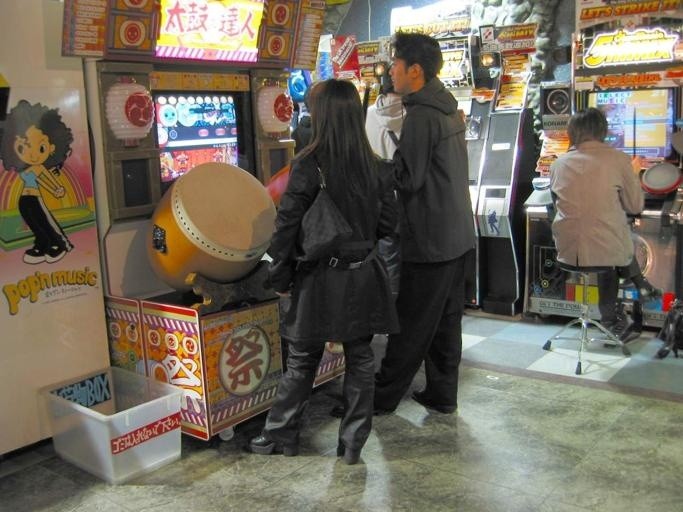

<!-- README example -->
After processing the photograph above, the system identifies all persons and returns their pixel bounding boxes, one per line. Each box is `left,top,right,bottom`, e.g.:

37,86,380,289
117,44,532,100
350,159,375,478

365,65,405,158
333,33,476,414
250,80,400,465
549,108,655,326
291,84,315,151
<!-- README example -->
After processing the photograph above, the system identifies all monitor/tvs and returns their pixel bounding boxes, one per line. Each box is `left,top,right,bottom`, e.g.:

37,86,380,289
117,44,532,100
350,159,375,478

151,88,257,198
586,87,675,159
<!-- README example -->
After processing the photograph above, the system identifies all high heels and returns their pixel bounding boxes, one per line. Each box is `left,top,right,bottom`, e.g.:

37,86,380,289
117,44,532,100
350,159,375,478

248,432,300,458
336,440,363,466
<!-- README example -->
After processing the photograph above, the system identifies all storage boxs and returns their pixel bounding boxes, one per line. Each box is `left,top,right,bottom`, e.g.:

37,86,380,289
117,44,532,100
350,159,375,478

35,365,186,484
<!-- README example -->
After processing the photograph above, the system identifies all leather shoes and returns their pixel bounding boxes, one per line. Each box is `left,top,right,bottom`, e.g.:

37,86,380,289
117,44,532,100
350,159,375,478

331,402,393,417
412,389,457,413
638,282,664,303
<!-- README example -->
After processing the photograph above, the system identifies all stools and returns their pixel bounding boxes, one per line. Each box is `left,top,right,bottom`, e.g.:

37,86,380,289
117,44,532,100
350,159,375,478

541,259,631,376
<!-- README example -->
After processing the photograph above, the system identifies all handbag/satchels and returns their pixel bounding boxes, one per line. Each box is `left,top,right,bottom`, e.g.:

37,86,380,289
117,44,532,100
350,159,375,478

292,187,356,268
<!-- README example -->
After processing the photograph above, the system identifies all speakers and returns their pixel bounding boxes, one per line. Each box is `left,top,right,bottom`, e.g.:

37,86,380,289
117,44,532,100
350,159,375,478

540,84,576,130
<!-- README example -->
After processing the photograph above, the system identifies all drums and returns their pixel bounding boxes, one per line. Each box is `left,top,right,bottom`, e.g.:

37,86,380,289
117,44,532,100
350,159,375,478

146,162,277,289
643,163,679,192
